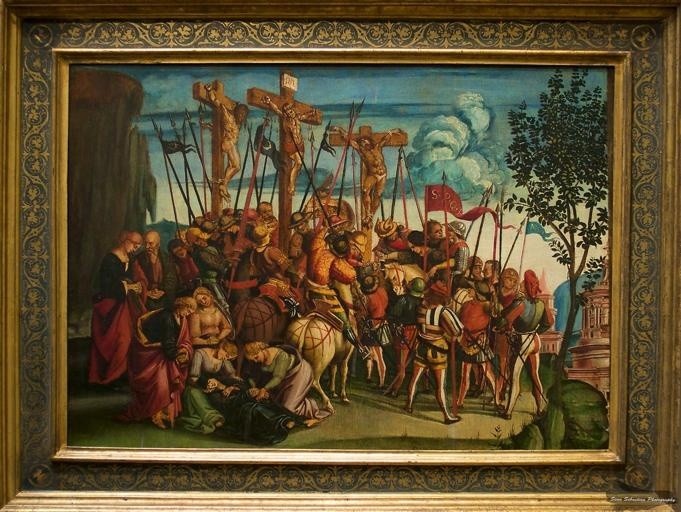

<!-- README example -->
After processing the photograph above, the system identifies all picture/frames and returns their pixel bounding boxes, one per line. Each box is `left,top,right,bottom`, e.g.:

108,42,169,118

0,0,681,509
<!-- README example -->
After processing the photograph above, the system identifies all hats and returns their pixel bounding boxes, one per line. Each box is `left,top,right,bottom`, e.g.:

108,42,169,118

323,215,350,230
287,211,313,230
429,279,447,298
523,267,541,300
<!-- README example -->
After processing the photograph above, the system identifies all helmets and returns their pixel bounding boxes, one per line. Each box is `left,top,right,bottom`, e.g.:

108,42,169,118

499,267,520,281
329,236,349,258
360,274,379,292
217,216,236,231
468,256,483,267
343,228,368,254
201,220,218,233
185,227,203,244
252,224,270,249
402,277,427,298
372,215,398,238
441,220,466,237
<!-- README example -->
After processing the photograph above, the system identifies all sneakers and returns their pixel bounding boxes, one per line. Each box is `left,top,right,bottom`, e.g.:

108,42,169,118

149,412,167,431
449,403,464,409
536,407,546,416
495,405,512,420
403,406,412,413
365,377,401,398
444,415,462,425
